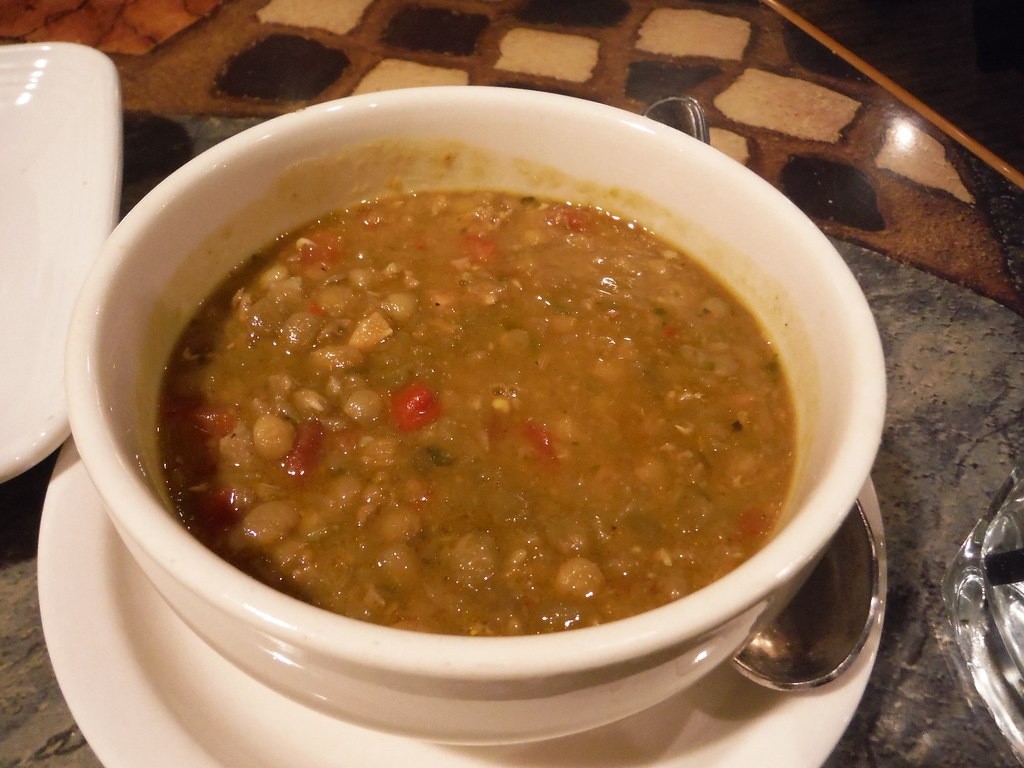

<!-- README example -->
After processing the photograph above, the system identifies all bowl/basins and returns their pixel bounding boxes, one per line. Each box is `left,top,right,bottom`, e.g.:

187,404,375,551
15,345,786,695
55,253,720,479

65,86,888,747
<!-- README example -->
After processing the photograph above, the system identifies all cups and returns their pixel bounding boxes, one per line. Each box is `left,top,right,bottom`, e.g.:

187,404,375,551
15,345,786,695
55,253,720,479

928,465,1024,768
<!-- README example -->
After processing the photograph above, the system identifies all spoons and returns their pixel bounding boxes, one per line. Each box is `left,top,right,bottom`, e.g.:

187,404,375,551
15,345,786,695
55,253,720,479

641,97,879,694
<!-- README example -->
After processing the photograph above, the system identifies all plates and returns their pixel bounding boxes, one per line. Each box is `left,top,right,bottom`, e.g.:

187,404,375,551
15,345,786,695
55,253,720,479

0,41,122,484
37,441,886,768
